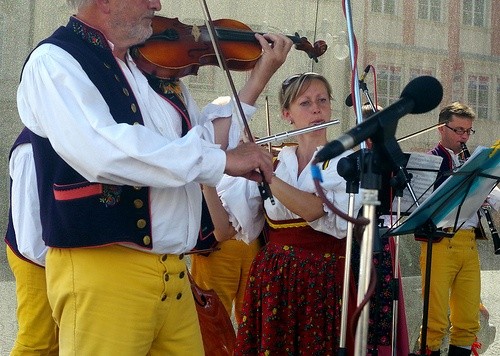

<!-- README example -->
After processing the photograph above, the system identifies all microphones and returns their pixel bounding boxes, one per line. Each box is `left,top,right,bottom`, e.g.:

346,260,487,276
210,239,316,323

316,76,444,162
345,64,371,107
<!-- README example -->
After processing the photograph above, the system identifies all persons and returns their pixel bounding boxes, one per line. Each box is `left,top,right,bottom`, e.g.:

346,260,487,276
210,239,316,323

3,126,59,356
189,72,409,356
17,0,295,356
414,101,500,356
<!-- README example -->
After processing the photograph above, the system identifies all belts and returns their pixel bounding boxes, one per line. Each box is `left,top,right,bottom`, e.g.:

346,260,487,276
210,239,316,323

437,227,474,232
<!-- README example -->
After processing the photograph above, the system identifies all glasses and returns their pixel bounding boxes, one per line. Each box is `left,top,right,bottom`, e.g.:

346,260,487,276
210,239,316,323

444,125,476,136
282,72,320,99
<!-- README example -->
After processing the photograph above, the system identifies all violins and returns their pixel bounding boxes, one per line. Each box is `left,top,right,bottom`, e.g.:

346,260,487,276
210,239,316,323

128,15,328,82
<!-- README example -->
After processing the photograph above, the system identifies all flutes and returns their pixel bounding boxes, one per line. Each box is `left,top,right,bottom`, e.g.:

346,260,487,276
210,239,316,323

460,142,500,255
254,118,340,146
